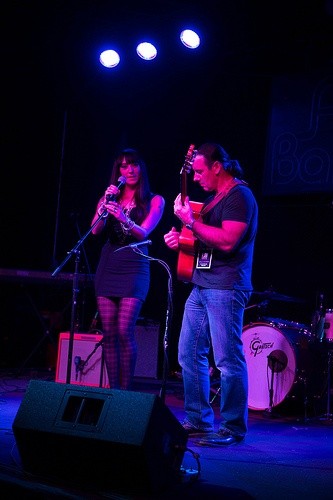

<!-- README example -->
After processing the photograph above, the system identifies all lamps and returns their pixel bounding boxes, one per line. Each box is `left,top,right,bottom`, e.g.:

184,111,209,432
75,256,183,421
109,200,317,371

177,17,208,52
96,40,120,69
135,33,161,60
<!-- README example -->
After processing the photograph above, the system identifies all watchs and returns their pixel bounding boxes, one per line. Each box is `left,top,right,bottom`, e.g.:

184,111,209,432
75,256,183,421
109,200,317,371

186,218,195,229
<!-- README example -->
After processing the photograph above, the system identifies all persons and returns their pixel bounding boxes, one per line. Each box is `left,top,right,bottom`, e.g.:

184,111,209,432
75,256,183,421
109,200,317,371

164,142,260,449
91,149,165,390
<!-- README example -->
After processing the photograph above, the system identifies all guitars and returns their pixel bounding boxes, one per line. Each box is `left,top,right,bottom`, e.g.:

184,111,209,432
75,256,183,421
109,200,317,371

175,144,205,281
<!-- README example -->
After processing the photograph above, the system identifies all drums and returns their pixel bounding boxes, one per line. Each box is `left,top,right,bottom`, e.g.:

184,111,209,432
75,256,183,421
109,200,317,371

256,283,326,343
324,309,333,343
242,320,329,412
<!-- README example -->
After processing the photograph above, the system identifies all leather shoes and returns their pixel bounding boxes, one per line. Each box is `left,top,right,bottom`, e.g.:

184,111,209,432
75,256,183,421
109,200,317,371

194,430,245,447
181,423,214,433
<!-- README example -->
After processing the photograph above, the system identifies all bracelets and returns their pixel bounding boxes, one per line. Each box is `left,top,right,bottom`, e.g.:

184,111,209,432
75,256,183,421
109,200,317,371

121,219,135,231
97,207,109,219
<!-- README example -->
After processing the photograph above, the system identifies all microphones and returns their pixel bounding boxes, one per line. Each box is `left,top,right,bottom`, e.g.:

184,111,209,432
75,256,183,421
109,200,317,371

266,356,285,365
113,240,152,253
74,355,80,372
106,175,127,199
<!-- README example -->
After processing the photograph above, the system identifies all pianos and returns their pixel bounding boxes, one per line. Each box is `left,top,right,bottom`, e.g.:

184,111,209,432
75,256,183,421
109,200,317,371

0,267,99,377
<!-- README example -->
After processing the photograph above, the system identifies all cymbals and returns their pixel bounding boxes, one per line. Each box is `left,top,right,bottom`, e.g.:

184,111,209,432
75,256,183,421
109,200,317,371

252,290,299,301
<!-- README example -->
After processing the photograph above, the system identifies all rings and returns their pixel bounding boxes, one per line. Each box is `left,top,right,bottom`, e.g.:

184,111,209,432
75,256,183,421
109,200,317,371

113,208,117,212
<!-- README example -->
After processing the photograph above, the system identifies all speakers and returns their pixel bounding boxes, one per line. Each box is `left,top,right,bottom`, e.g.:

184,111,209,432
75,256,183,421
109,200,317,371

55,330,110,390
12,378,190,498
